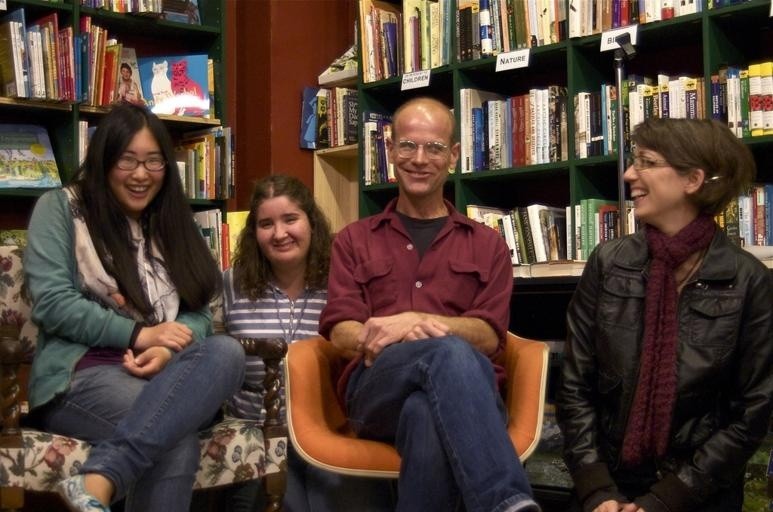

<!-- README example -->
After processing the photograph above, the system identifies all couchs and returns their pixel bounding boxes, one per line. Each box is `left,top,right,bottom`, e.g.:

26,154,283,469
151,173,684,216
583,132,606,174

0,246,289,512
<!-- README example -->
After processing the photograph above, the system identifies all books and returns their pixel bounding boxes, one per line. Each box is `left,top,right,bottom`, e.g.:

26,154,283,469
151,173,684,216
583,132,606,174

296,1,773,186
1,0,217,120
466,197,640,265
0,124,62,190
177,125,236,272
712,186,773,249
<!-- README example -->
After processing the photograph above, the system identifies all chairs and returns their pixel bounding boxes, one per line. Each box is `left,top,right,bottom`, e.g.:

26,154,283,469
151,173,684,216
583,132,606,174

284,329,550,480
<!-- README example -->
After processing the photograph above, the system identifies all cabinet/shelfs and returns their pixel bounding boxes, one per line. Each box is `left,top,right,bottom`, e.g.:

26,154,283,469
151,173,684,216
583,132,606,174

313,146,359,237
357,0,773,281
0,0,236,269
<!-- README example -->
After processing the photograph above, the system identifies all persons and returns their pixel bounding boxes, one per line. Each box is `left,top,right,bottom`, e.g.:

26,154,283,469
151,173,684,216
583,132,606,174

319,95,542,511
211,176,328,511
553,117,773,512
25,102,246,512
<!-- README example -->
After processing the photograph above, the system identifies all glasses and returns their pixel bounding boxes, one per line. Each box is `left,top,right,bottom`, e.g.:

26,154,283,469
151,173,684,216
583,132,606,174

392,140,453,161
625,152,674,171
114,153,169,173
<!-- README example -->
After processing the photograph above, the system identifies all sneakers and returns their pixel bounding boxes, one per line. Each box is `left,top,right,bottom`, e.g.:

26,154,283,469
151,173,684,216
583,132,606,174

55,472,114,512
